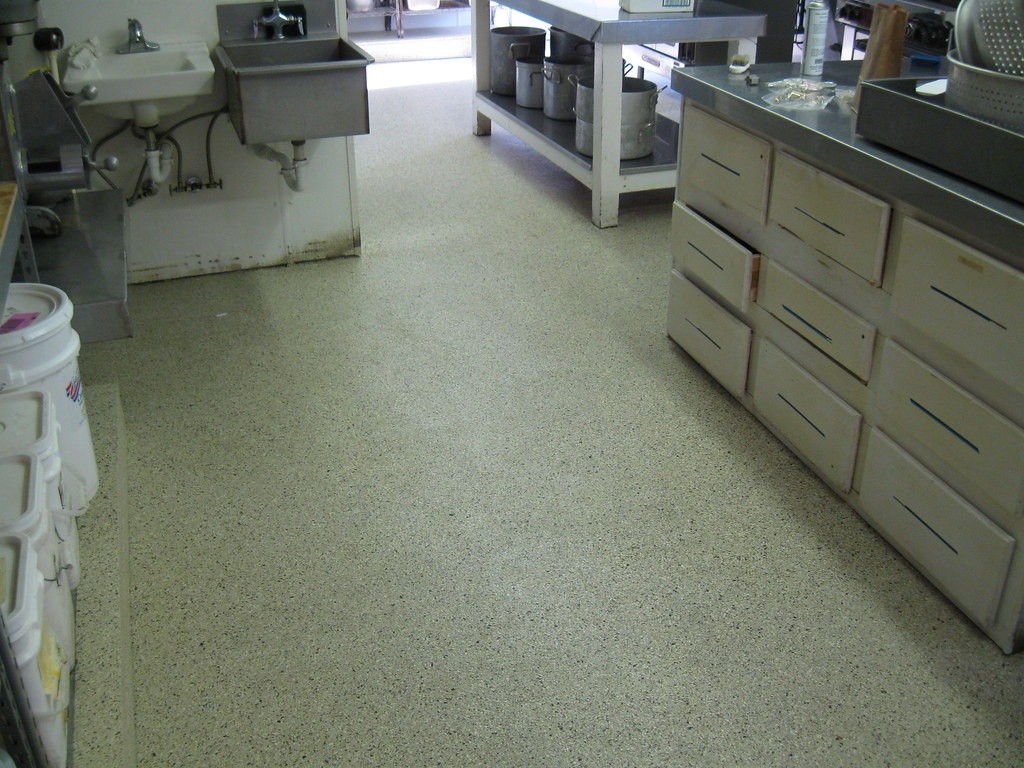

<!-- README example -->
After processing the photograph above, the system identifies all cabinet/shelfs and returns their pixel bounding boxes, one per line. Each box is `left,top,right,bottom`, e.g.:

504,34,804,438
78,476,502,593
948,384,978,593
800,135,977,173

666,60,1024,653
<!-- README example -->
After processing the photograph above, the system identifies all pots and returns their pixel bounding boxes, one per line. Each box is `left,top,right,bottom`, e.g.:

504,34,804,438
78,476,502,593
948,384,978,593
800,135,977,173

515,61,544,108
568,73,668,158
543,56,634,121
489,26,547,96
549,25,595,62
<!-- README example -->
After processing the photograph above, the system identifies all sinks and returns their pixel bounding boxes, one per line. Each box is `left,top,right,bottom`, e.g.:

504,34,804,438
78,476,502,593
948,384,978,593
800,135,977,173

218,31,378,148
61,42,215,106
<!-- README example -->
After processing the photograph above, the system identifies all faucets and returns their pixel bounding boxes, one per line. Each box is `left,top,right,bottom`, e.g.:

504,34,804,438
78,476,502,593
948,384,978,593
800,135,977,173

125,15,149,52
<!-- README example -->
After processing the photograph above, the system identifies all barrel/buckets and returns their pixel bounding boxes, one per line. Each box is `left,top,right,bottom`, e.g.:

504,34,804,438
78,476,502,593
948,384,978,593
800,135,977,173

1,281,99,510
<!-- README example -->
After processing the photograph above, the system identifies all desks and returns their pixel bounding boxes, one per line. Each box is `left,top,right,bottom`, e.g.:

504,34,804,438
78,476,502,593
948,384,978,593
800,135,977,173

471,0,767,229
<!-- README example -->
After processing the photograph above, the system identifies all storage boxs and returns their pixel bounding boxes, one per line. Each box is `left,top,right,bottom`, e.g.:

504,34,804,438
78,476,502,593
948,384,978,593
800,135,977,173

0,387,80,768
855,76,1024,204
